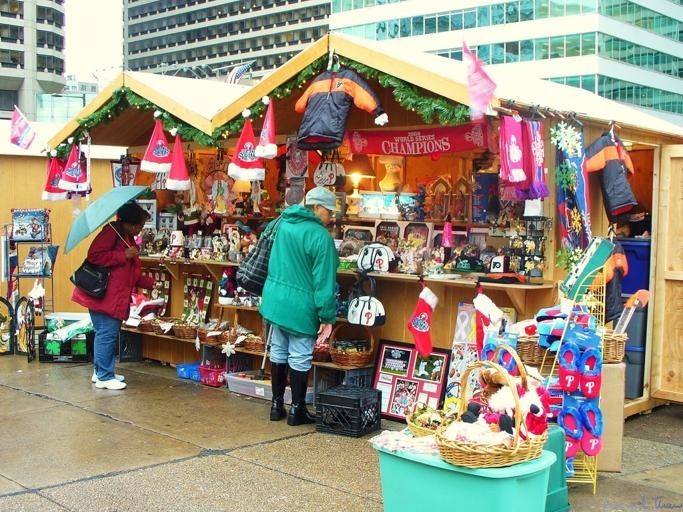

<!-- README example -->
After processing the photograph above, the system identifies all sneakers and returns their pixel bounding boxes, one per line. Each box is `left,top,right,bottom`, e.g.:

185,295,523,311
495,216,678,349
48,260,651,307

481,338,520,376
557,402,602,459
558,342,602,399
91,373,124,382
95,379,127,390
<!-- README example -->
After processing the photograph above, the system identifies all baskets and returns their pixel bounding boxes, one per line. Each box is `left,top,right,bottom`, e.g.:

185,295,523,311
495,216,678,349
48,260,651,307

139,317,331,362
316,366,381,438
176,344,251,387
405,361,549,469
39,329,141,363
517,328,630,366
329,323,375,367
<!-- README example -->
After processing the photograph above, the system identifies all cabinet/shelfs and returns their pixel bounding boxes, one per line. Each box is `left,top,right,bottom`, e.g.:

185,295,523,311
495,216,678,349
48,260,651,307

6,222,54,355
211,299,380,372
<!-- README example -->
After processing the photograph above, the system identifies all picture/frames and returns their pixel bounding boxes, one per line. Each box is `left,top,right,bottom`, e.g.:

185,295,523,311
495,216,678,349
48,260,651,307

365,337,450,424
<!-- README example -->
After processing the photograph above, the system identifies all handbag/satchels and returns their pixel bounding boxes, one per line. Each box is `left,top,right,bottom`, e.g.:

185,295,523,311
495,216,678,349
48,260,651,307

314,161,346,186
237,239,274,297
348,296,386,327
357,244,396,272
70,259,108,298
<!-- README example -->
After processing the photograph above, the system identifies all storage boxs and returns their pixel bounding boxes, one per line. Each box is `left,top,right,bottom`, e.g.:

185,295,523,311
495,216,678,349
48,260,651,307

606,238,652,397
372,417,556,512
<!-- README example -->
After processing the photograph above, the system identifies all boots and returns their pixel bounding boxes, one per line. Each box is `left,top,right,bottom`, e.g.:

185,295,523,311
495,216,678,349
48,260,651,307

288,363,316,426
270,362,288,420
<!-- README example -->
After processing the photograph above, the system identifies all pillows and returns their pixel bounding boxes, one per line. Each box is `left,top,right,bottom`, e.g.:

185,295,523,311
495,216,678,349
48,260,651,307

10,207,59,277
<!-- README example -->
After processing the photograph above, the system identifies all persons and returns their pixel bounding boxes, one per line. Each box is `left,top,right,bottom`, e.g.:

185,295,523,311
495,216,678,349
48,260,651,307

67,200,162,394
255,184,340,427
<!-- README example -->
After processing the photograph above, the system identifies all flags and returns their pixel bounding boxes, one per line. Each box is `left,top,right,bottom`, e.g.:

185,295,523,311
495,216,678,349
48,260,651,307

9,103,35,150
459,37,497,121
223,61,254,85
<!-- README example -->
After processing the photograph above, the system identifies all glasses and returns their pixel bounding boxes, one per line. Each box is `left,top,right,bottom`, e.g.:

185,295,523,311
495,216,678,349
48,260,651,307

320,205,336,218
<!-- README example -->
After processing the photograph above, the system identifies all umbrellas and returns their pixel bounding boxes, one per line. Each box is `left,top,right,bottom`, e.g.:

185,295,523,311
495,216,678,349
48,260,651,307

61,184,149,256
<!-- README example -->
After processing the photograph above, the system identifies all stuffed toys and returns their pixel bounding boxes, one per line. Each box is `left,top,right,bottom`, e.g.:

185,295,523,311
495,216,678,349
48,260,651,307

445,362,552,445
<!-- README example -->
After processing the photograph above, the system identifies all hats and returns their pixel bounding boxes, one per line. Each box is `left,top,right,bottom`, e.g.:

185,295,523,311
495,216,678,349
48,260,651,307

254,96,278,160
165,128,191,192
306,186,341,211
58,137,88,192
42,150,68,201
140,119,172,173
227,108,265,181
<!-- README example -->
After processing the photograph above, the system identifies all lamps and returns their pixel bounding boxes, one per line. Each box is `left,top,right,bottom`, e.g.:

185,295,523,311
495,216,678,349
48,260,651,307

340,152,376,217
